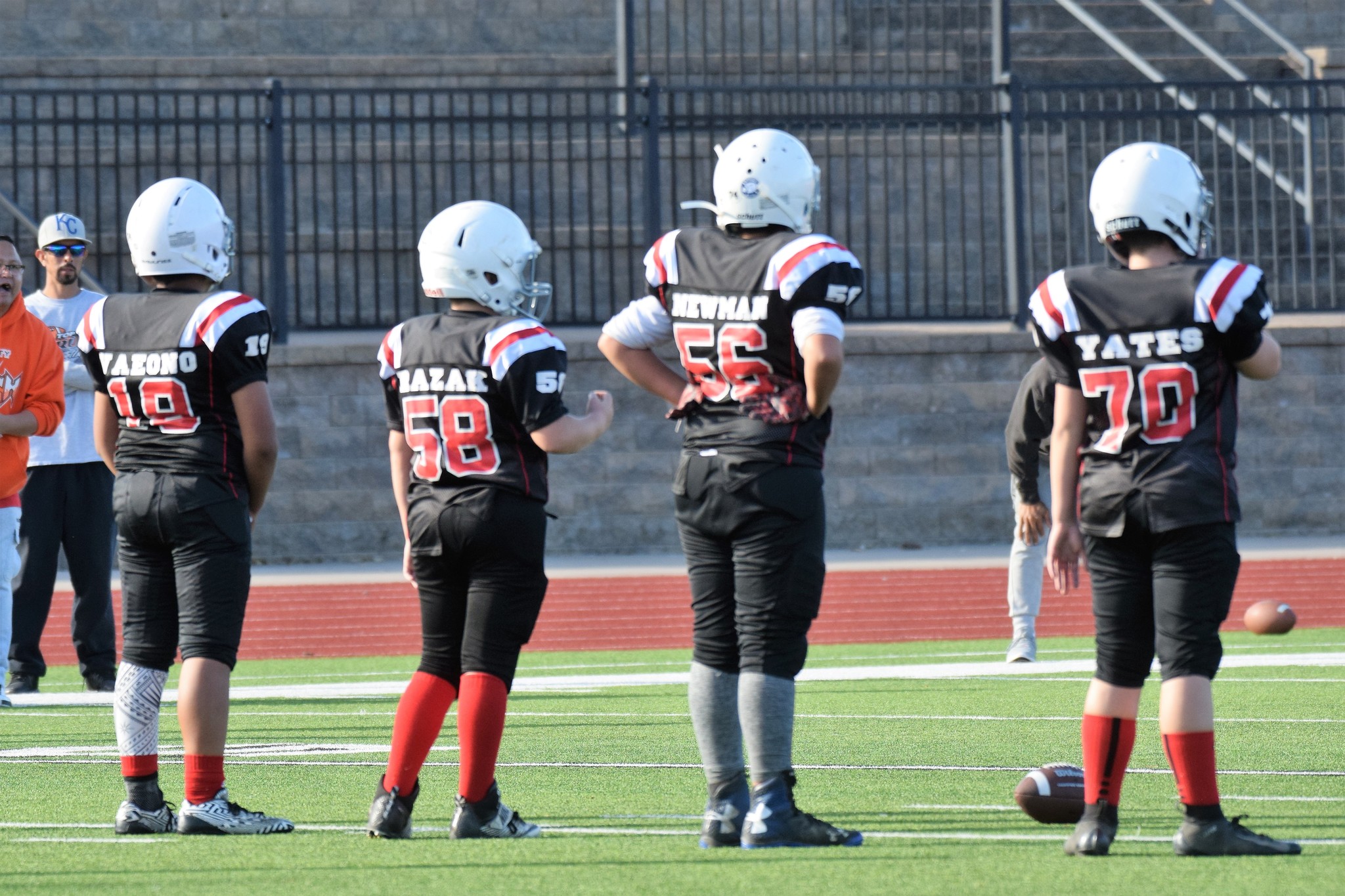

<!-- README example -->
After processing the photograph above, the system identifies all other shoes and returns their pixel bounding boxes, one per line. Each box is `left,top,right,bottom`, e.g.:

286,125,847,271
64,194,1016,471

0,688,13,709
1005,639,1038,664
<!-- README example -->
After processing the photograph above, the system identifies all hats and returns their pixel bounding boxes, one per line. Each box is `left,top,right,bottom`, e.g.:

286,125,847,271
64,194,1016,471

38,213,92,251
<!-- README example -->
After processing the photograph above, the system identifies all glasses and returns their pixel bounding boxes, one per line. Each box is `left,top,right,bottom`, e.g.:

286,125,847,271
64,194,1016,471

42,243,86,257
0,263,26,277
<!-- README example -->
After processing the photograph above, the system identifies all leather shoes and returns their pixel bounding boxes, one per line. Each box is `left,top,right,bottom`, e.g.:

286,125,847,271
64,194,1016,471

4,670,39,694
86,673,117,693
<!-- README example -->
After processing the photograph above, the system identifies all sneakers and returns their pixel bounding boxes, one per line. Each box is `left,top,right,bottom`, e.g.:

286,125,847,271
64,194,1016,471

448,776,541,838
365,772,420,838
700,773,751,847
113,799,181,835
1065,798,1120,854
741,767,862,849
175,788,294,833
1171,811,1301,856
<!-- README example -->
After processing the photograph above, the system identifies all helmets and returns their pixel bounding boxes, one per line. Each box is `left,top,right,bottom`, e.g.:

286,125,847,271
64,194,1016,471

416,200,553,325
1087,142,1215,261
126,177,235,286
713,127,821,236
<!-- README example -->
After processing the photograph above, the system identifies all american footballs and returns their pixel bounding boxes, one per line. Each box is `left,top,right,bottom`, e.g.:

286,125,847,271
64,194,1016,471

1014,764,1088,823
1242,601,1297,634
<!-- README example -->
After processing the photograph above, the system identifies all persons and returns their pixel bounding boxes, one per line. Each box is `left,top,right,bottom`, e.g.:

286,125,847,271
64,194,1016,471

1,237,68,710
1041,143,1300,858
596,127,863,848
11,212,120,694
1004,352,1077,668
76,176,298,836
365,198,616,840
